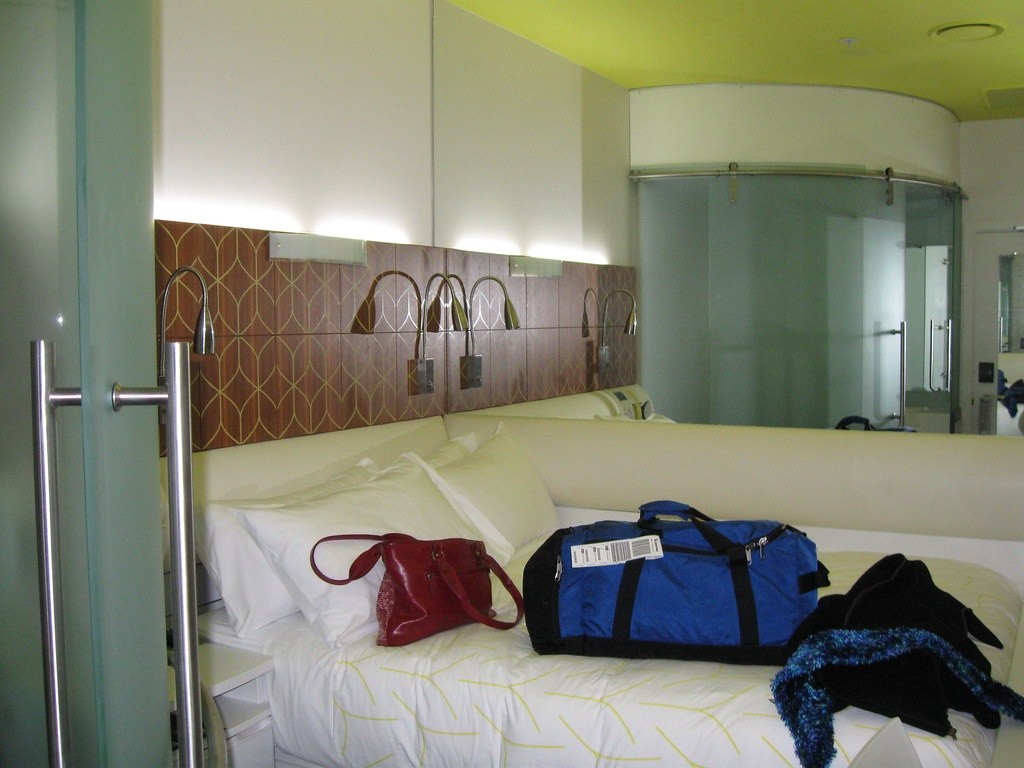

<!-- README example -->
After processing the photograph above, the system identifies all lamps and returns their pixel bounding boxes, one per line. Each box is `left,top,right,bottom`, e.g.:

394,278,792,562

599,289,637,371
415,272,470,392
460,275,521,388
158,265,216,423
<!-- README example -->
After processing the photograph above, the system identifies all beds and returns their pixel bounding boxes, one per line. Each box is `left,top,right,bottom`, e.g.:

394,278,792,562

198,548,1024,768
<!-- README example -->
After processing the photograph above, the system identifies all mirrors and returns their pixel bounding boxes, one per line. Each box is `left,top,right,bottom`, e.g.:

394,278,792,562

430,0,1024,434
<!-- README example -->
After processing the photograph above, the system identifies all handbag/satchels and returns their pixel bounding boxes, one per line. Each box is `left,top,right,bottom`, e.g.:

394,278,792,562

310,533,524,647
523,499,830,666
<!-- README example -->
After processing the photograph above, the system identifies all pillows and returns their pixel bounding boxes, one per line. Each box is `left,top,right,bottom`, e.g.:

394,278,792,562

647,413,676,422
439,418,559,550
195,455,381,637
229,452,516,648
423,430,478,470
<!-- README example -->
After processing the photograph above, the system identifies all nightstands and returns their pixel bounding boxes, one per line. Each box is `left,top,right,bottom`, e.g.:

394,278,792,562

167,642,276,768
